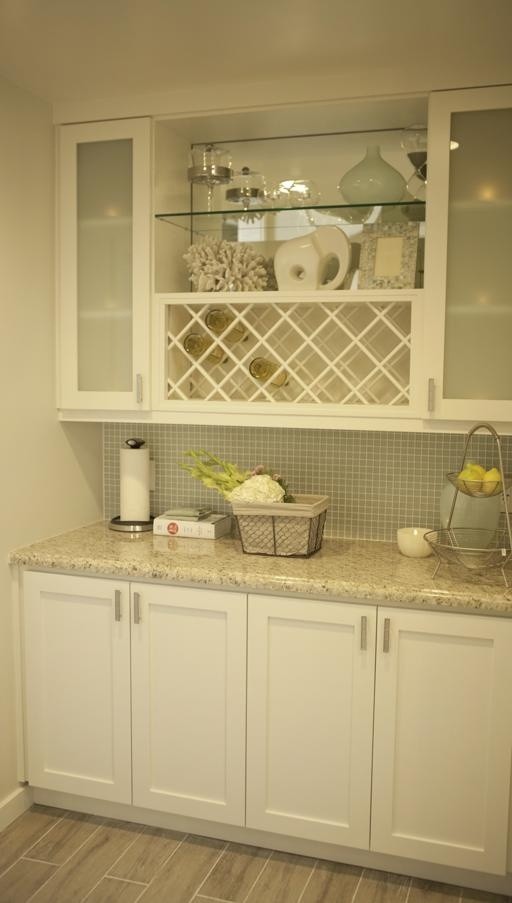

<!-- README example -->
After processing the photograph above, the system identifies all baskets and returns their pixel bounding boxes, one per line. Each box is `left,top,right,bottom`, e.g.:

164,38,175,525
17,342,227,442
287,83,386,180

447,471,512,497
233,493,330,558
424,526,512,569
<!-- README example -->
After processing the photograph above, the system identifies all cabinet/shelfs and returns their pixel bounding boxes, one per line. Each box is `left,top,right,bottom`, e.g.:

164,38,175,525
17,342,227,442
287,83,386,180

48,88,159,430
10,570,243,847
421,83,510,435
158,87,420,433
244,593,512,899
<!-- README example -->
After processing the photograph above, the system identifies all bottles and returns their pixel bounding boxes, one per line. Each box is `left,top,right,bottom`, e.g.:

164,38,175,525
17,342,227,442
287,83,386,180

183,333,230,364
439,480,501,557
205,309,248,344
339,142,406,206
248,356,292,387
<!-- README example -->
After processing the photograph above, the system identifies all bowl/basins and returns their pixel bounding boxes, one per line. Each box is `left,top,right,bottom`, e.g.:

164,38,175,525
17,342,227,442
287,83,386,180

396,526,437,559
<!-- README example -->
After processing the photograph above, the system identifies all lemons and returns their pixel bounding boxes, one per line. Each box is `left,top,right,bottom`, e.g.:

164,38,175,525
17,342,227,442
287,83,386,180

466,462,486,479
482,467,502,493
458,466,483,490
